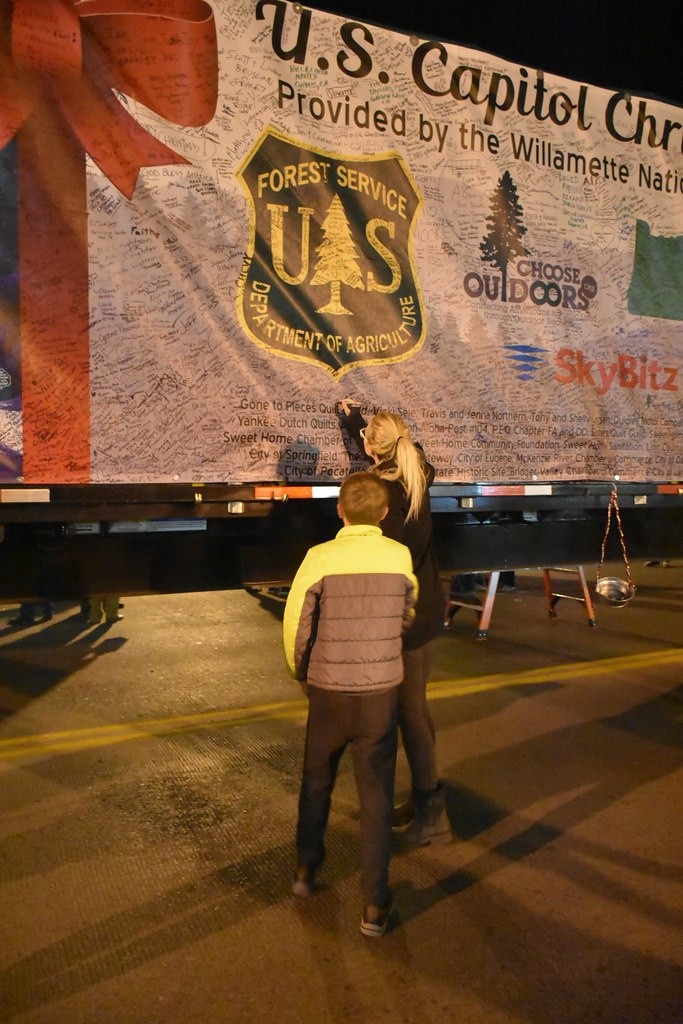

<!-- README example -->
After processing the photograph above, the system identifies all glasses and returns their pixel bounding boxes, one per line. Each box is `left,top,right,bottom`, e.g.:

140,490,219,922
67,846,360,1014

359,427,367,442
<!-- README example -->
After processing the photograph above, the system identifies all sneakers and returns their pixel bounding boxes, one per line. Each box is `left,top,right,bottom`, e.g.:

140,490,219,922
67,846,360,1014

291,864,315,897
360,891,395,937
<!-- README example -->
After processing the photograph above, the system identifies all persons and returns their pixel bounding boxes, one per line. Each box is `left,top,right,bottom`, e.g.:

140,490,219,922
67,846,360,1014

282,472,418,938
334,398,453,851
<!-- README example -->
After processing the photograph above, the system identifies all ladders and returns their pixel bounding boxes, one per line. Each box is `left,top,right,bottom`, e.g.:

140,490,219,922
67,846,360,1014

436,563,598,646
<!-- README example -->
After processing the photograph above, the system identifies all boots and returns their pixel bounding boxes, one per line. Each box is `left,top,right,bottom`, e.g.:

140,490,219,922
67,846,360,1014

389,781,453,846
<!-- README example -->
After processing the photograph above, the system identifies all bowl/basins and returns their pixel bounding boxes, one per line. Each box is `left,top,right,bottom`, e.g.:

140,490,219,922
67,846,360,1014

595,577,635,608
